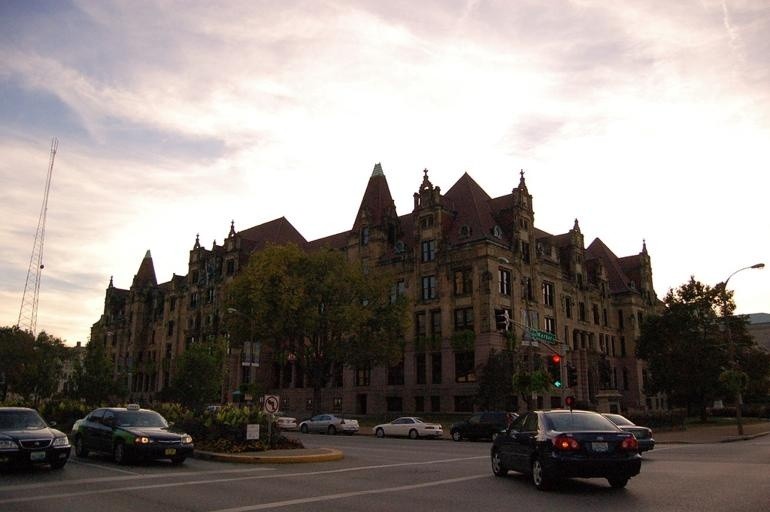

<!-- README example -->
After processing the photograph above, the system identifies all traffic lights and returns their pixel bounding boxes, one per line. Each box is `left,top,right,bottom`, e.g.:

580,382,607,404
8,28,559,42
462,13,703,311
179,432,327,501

566,363,578,388
551,355,561,390
495,309,509,332
565,396,574,405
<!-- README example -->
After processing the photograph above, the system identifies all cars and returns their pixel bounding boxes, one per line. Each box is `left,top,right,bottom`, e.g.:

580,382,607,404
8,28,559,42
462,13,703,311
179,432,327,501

72,403,194,465
451,411,519,441
299,413,359,435
275,411,297,431
490,410,642,487
599,411,655,454
0,406,71,473
372,416,443,439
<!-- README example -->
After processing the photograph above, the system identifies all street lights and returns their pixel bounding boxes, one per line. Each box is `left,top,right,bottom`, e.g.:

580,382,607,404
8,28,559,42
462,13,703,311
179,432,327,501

227,307,253,382
723,262,765,434
